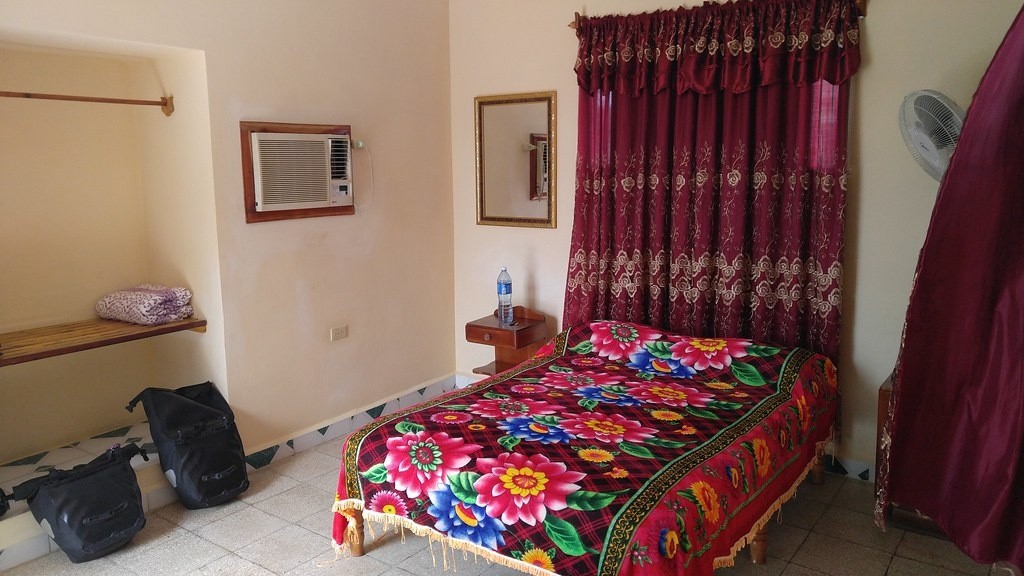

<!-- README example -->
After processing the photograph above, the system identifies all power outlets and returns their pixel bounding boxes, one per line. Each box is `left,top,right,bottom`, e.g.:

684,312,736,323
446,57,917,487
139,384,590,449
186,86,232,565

524,143,529,151
353,139,365,150
331,325,349,342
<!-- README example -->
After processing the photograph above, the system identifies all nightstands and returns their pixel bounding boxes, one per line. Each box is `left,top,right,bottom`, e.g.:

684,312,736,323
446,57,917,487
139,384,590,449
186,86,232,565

465,305,546,376
874,372,953,540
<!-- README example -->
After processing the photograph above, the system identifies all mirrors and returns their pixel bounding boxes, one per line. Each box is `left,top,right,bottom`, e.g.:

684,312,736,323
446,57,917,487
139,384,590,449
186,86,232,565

474,90,557,228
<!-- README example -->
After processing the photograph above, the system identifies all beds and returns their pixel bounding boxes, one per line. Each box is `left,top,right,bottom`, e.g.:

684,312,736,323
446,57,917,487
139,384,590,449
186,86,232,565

331,319,843,576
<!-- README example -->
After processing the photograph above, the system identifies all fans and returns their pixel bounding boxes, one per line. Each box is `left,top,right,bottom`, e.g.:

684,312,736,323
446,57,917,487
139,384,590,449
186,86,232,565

898,90,966,182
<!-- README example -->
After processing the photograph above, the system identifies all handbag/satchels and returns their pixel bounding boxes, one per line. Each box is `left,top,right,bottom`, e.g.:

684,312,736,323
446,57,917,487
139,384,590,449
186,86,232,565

0,434,149,564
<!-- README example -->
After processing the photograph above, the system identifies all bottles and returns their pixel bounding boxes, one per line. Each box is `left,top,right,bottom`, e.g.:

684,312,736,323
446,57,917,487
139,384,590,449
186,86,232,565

496,267,514,323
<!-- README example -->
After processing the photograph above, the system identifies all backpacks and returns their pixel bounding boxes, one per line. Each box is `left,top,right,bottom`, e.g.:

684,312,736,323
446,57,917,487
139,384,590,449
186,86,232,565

126,382,251,510
